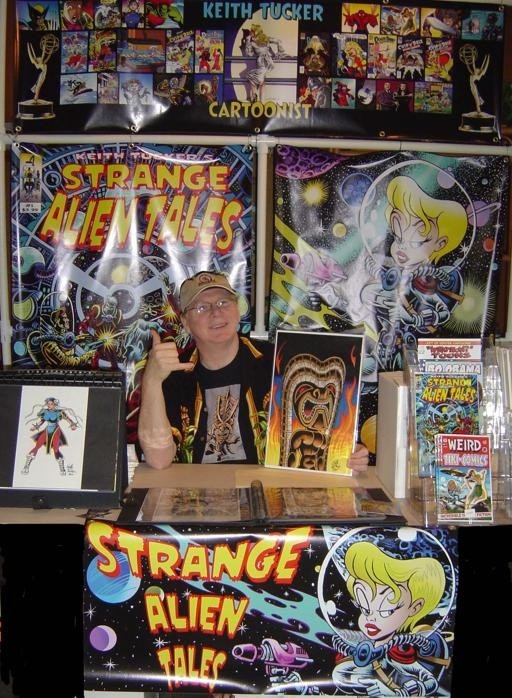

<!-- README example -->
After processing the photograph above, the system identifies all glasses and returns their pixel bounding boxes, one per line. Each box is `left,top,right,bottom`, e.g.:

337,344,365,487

182,295,238,319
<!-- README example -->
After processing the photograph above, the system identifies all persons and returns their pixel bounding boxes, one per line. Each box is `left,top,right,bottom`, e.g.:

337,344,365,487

137,271,369,479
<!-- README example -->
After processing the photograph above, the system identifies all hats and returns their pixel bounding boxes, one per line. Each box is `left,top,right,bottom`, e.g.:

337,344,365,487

180,272,238,313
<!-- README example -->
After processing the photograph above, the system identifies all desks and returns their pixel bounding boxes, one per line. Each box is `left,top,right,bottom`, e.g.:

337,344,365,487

0,505,510,698
